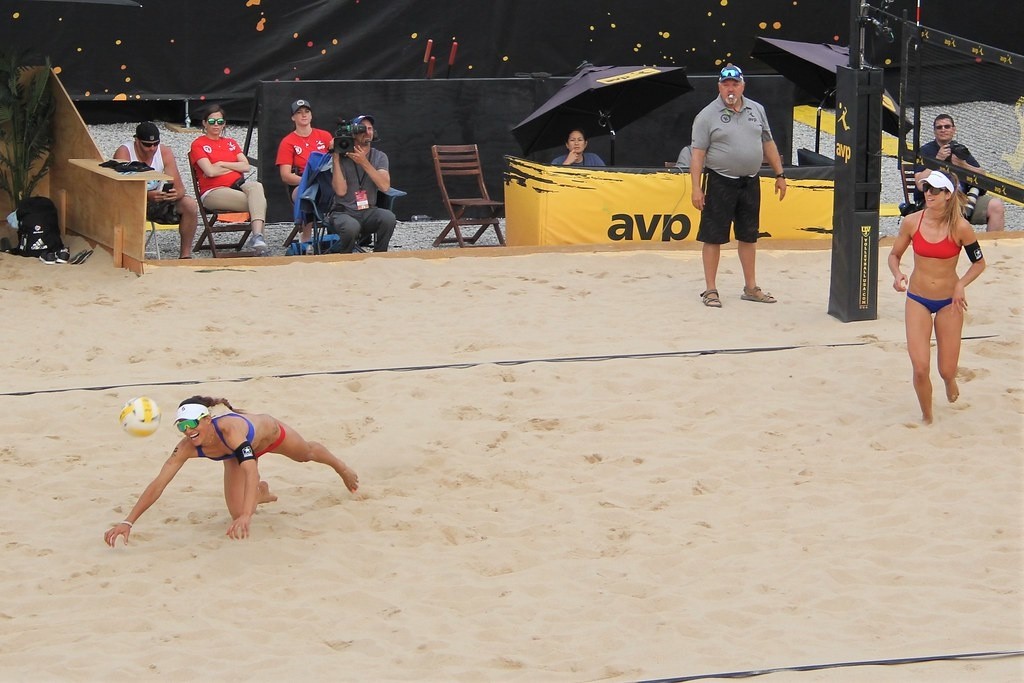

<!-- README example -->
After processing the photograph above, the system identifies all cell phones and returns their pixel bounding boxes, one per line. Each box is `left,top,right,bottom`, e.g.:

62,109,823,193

162,183,173,196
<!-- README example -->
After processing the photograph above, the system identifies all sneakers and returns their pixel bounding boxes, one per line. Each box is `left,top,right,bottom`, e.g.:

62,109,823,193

39,250,57,264
55,247,70,263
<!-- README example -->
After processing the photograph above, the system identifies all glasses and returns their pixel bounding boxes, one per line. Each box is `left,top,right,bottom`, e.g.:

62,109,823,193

923,183,943,195
141,141,160,147
207,118,225,125
177,412,209,432
935,124,954,130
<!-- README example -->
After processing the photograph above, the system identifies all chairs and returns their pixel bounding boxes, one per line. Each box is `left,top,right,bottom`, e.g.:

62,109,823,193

431,144,505,248
187,151,259,258
283,151,407,255
901,164,915,205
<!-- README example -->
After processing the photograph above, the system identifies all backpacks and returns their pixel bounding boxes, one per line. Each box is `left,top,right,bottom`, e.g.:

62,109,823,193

7,197,64,258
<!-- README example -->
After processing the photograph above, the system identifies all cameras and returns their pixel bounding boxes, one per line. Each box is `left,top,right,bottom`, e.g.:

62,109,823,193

947,142,969,160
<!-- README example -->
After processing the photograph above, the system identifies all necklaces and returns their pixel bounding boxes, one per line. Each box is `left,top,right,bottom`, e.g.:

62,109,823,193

305,143,309,147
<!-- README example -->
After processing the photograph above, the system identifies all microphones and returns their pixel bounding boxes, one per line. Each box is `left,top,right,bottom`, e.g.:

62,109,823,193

364,138,381,143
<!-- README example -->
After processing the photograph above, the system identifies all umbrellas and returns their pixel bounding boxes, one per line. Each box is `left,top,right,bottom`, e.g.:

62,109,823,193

751,36,913,153
510,60,694,166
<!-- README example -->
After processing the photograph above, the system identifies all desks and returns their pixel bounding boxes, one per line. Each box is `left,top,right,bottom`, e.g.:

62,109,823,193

67,158,175,181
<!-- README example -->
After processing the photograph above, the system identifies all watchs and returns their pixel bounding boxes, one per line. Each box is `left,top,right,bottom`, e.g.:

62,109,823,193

775,172,786,179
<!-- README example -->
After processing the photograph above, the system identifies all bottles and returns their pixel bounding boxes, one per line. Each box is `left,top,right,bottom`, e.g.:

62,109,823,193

306,241,314,255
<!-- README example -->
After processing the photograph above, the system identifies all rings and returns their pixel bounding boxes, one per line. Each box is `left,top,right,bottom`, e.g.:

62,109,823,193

352,156,355,158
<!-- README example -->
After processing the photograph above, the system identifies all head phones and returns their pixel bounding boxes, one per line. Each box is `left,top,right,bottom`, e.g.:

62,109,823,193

352,112,378,140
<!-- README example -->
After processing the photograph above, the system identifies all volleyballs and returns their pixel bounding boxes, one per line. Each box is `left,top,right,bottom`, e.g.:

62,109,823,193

119,395,162,438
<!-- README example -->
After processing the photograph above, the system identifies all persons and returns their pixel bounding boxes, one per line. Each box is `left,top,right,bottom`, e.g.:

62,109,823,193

104,395,359,548
552,127,605,167
690,65,786,307
914,113,1005,231
326,115,397,254
887,171,986,426
275,99,334,242
113,121,199,259
191,104,269,257
676,145,706,168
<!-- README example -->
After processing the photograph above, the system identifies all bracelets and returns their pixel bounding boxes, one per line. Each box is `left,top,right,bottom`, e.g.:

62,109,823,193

121,521,133,528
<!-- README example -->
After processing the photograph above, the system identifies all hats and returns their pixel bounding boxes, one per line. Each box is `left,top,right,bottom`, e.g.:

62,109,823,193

918,171,954,194
719,65,743,82
173,403,209,426
291,99,312,116
351,114,375,126
133,122,160,141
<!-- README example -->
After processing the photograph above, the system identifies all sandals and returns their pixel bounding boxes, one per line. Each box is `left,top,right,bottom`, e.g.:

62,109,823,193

741,286,777,303
700,289,722,308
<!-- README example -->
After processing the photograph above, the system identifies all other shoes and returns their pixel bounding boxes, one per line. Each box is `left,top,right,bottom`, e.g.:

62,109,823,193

247,234,276,257
